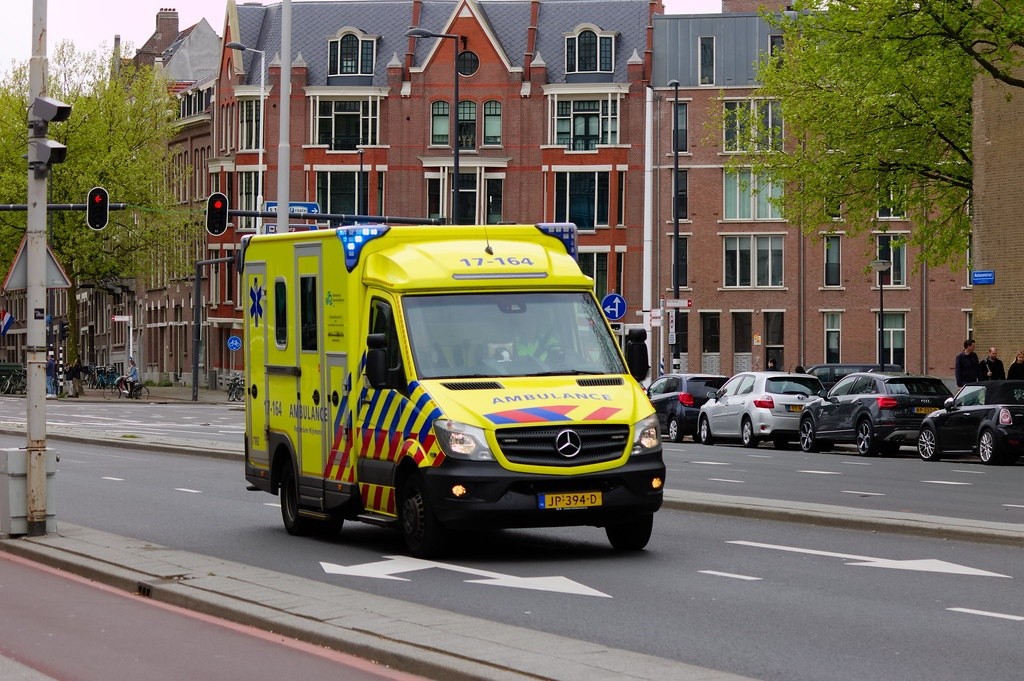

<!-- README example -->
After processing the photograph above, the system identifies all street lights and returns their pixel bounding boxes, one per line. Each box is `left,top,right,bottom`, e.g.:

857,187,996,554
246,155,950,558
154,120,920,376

225,42,264,233
870,259,893,372
403,27,459,225
666,79,680,374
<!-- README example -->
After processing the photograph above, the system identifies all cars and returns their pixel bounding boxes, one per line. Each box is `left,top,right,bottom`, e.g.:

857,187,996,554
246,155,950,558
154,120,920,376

645,373,731,443
696,369,826,450
916,379,1024,468
797,371,955,456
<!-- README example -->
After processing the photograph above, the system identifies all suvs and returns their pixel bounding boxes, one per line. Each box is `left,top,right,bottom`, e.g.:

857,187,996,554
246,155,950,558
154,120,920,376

782,361,905,401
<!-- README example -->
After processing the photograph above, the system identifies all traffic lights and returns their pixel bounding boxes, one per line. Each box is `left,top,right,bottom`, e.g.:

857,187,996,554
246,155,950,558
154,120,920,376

86,186,110,230
205,192,229,237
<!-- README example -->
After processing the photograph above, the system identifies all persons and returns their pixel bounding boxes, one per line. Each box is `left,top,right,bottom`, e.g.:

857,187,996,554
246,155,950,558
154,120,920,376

46,355,80,399
518,321,559,363
980,346,1008,382
764,358,781,371
955,339,980,392
121,360,138,399
1006,351,1024,381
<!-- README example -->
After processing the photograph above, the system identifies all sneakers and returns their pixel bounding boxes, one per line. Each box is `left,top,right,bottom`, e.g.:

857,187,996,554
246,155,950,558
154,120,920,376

46,394,58,400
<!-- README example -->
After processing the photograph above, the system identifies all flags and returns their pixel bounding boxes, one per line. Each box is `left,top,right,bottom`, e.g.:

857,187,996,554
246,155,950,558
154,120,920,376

0,308,15,339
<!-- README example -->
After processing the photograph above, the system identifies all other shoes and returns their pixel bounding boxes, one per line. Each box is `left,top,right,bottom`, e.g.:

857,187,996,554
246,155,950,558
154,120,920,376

74,392,79,398
123,390,130,394
68,394,75,398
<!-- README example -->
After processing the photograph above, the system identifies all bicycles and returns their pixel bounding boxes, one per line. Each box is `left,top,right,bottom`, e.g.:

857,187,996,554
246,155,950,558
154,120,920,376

0,364,150,403
226,376,246,403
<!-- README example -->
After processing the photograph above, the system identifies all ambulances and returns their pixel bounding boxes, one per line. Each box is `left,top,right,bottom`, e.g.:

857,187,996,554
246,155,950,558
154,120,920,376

238,220,668,560
